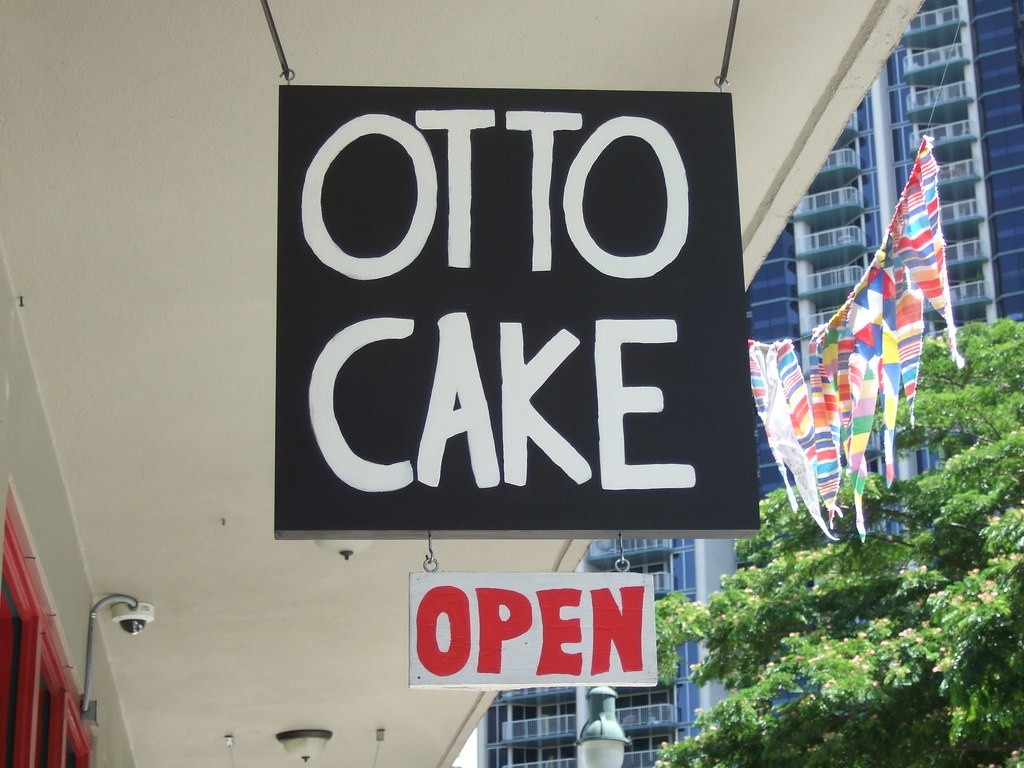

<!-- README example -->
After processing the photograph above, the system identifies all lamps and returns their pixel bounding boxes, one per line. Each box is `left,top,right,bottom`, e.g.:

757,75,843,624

573,687,632,768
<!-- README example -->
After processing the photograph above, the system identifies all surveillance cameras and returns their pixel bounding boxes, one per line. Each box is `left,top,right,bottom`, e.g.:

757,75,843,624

110,603,155,636
